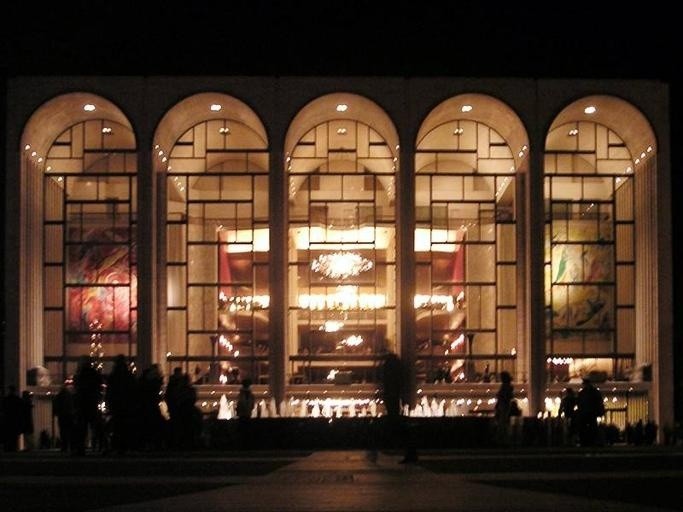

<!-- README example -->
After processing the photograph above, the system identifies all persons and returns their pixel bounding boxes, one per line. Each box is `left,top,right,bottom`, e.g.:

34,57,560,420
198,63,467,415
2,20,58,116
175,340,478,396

557,388,580,453
363,352,419,463
226,367,242,384
622,419,681,450
1,354,198,457
493,371,513,451
576,380,605,456
236,380,255,419
